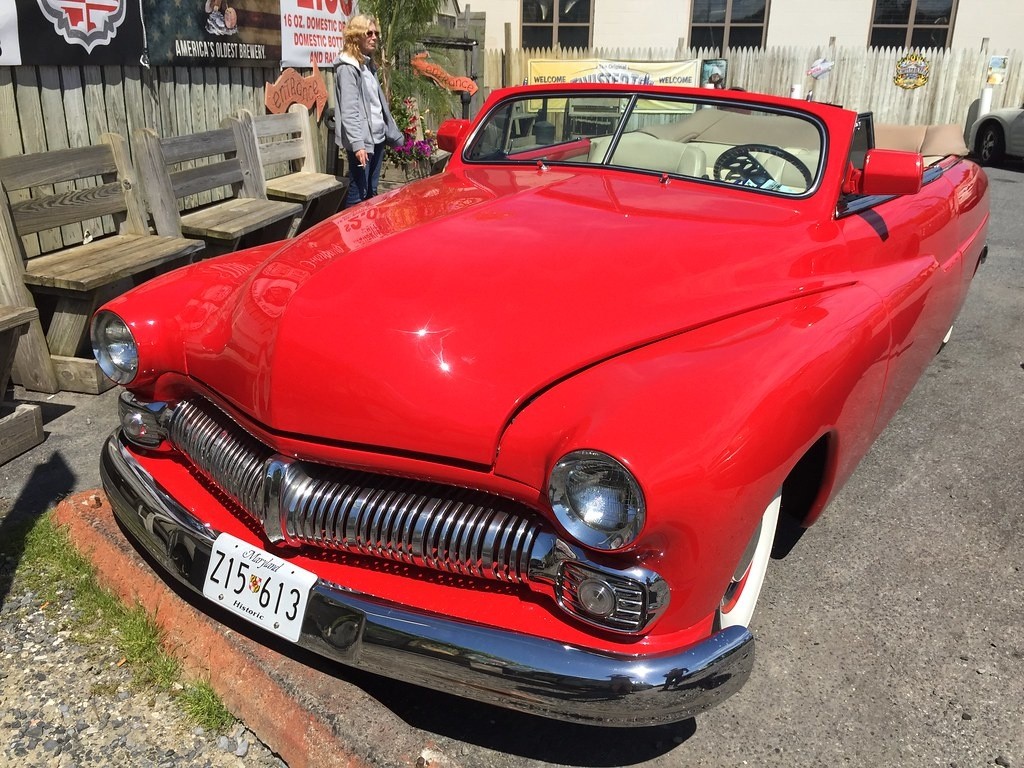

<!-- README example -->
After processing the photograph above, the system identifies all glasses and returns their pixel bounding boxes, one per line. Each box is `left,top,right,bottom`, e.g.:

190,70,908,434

367,31,380,38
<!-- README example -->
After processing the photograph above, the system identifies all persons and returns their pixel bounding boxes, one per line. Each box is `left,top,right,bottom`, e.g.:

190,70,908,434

703,64,724,89
333,15,404,208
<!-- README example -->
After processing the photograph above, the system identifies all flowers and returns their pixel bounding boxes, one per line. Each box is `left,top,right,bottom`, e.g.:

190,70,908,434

381,96,441,179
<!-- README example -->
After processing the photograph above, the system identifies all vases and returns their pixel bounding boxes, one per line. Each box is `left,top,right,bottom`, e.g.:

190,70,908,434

377,150,453,184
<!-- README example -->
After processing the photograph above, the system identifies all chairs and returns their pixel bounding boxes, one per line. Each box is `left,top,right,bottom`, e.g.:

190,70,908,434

588,136,707,180
761,155,821,193
480,89,538,147
0,103,351,467
566,97,621,140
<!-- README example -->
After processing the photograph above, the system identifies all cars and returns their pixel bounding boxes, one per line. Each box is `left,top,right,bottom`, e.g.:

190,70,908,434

967,102,1024,165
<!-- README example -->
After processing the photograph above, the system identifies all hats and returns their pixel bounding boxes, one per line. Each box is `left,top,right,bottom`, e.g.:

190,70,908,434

711,66,721,76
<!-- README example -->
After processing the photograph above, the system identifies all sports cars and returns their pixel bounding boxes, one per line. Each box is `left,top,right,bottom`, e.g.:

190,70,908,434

87,82,993,728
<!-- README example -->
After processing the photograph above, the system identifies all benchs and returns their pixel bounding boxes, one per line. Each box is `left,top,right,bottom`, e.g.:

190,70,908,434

683,141,822,183
